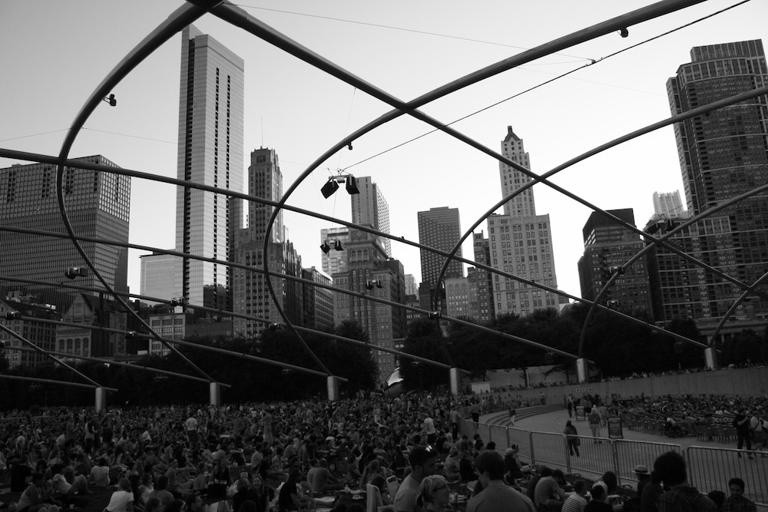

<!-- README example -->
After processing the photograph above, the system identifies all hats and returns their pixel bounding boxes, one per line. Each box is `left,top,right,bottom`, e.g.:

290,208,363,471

632,465,652,475
